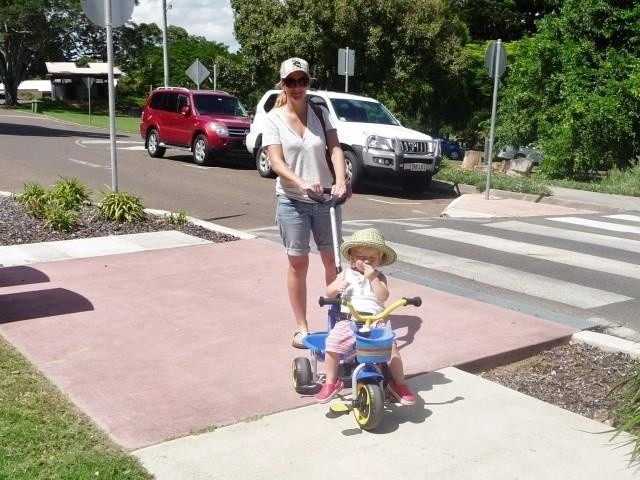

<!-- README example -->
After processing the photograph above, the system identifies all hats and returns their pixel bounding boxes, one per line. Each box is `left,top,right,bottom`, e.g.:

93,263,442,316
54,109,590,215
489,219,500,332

340,229,397,267
280,57,309,79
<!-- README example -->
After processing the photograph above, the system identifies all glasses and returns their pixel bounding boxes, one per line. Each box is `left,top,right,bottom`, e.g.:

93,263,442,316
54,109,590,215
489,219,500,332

284,76,309,88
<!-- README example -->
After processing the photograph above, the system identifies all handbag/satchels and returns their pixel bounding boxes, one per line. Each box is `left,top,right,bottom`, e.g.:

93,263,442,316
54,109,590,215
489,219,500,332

325,149,352,204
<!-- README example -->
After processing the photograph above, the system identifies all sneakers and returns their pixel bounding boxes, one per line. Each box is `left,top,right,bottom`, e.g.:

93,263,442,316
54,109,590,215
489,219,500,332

387,380,416,406
317,377,344,404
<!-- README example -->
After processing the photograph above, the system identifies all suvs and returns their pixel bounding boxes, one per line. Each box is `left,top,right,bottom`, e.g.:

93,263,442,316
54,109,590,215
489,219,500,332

247,87,441,203
137,82,253,168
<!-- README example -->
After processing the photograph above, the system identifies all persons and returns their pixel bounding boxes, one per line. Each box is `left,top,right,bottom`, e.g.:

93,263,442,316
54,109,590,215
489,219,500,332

316,225,418,406
262,58,350,350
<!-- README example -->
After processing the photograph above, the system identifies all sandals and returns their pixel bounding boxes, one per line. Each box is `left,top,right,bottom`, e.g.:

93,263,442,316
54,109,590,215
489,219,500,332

292,329,311,349
